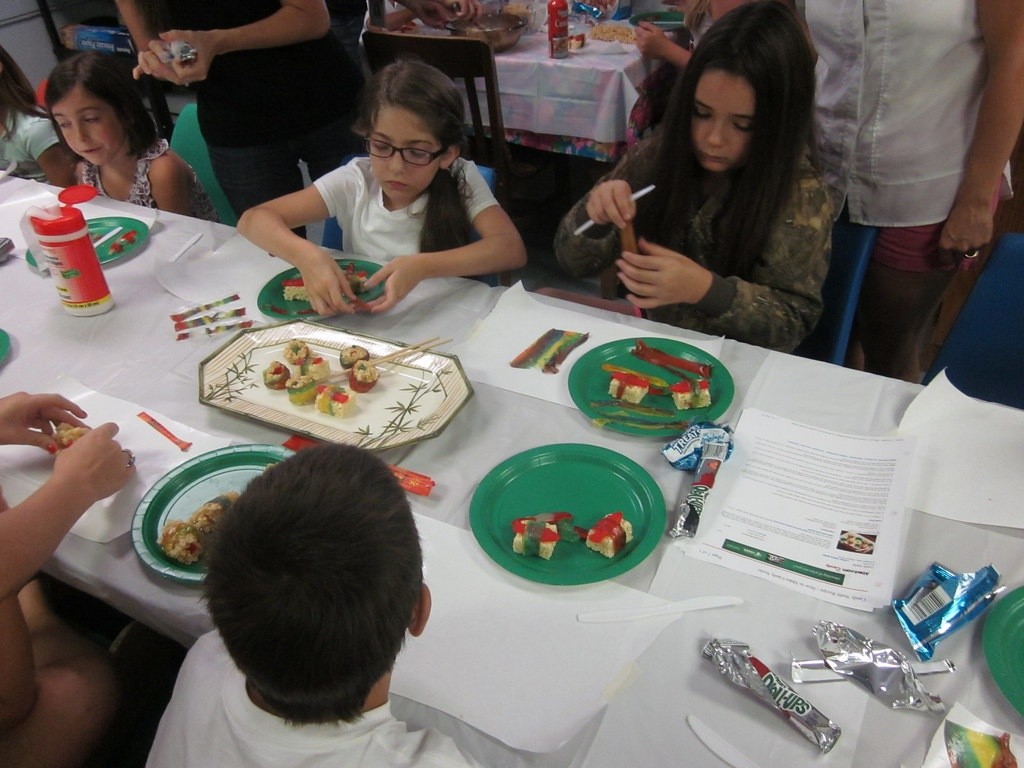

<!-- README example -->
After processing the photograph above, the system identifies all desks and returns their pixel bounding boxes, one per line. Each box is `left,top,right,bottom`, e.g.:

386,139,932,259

1,168,1023,767
355,21,685,164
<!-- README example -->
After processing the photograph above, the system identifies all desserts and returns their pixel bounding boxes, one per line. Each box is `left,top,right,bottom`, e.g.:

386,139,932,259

585,512,633,559
51,421,91,449
671,379,711,411
264,340,380,417
512,516,561,560
282,270,371,301
162,491,239,566
567,34,585,49
607,371,650,404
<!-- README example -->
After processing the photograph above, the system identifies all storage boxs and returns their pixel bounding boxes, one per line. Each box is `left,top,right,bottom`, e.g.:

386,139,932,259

60,23,131,54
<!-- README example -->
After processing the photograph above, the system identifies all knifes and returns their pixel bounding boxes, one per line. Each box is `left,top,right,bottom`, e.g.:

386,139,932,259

577,595,744,622
168,232,203,262
687,714,761,767
573,184,656,236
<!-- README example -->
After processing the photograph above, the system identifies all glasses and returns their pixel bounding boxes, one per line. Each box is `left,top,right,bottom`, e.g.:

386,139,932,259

363,128,449,165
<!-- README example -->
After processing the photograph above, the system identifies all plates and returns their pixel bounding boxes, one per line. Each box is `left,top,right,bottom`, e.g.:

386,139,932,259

132,444,295,584
0,328,10,365
568,337,735,436
983,584,1024,718
25,216,150,270
629,12,685,31
470,443,666,585
198,316,474,452
257,258,387,321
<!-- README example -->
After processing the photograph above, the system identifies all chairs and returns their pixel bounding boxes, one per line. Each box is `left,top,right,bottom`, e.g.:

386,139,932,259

918,233,1024,409
801,195,878,366
156,104,231,228
356,31,542,287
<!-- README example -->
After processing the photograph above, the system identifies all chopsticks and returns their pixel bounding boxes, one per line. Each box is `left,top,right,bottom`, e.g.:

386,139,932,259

315,337,454,386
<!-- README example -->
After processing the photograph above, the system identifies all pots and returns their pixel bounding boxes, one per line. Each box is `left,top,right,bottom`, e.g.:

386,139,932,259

445,11,528,53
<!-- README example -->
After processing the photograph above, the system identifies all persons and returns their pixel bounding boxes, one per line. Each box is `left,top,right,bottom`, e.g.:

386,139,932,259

44,49,221,223
113,0,372,239
0,392,137,768
326,0,482,76
0,46,77,188
634,0,746,70
144,442,479,768
802,0,1024,385
236,57,528,315
532,0,832,353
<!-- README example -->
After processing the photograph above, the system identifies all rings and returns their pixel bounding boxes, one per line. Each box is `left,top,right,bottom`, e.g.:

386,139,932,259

124,449,135,468
964,250,978,258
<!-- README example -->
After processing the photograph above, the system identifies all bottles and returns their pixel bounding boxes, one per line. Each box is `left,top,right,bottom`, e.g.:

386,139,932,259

547,0,569,59
